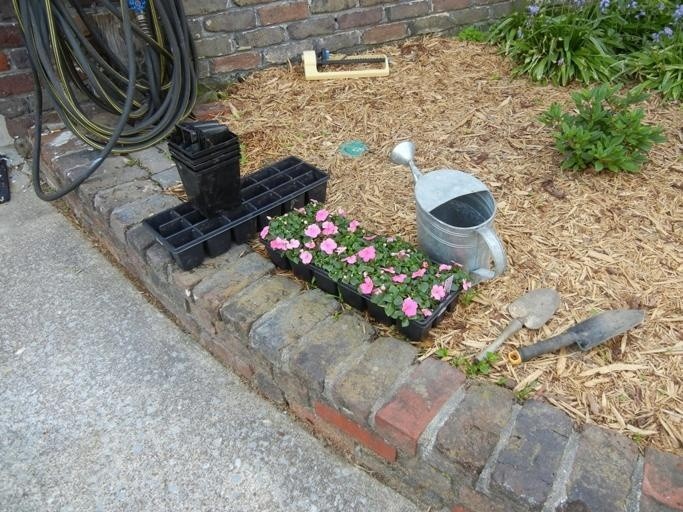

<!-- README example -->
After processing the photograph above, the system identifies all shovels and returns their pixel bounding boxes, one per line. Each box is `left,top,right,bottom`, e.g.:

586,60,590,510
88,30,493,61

473,289,644,365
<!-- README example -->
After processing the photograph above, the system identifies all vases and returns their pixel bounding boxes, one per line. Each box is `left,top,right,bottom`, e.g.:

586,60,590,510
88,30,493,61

260,236,464,344
140,118,330,272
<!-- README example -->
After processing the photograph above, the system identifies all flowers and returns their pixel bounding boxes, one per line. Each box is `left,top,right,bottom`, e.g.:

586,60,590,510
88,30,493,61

258,197,479,329
